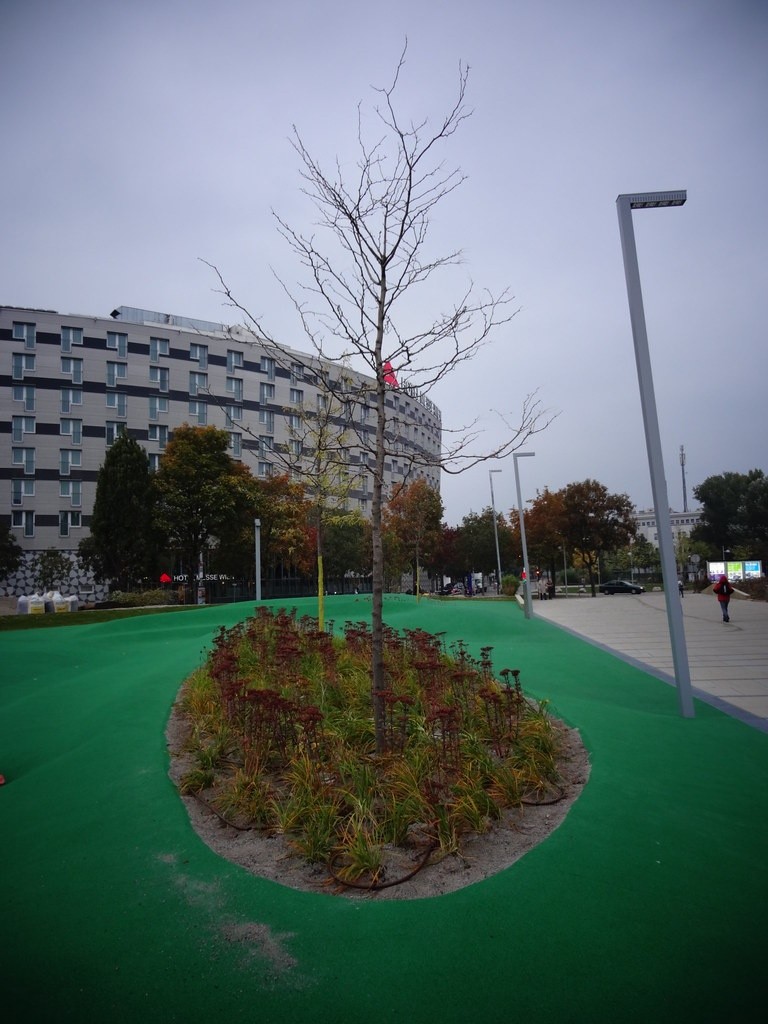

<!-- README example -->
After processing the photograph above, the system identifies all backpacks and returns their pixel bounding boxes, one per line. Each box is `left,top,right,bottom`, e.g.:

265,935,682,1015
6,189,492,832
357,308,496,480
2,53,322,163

719,581,730,595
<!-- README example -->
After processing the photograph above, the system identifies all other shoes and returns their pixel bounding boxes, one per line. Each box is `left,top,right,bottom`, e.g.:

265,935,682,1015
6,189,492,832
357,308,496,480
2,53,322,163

723,617,729,622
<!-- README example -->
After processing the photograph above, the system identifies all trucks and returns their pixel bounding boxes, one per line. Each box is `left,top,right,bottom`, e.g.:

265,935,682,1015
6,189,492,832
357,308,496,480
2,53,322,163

470,572,488,593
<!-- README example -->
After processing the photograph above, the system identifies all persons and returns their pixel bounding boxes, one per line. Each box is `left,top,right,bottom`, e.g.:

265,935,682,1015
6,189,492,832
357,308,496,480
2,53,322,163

545,576,557,600
476,580,485,595
713,575,734,622
536,575,547,600
676,578,685,598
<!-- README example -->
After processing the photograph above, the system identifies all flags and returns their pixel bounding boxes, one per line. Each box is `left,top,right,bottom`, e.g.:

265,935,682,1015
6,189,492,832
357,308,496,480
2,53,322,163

382,361,400,387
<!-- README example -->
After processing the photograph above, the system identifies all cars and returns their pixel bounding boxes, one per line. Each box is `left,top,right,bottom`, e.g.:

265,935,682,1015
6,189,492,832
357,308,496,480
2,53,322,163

599,579,644,595
521,565,540,581
437,584,476,596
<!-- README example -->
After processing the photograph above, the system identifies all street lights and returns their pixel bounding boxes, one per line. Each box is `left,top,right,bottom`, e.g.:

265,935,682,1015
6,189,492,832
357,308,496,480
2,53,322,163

618,188,694,723
723,546,730,561
513,452,537,620
489,469,503,596
